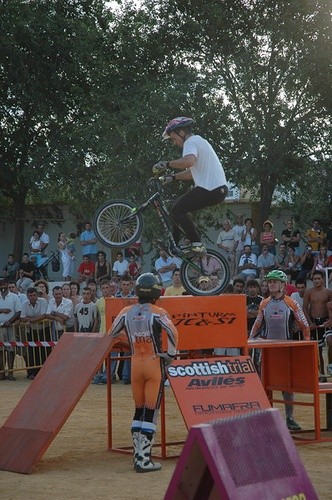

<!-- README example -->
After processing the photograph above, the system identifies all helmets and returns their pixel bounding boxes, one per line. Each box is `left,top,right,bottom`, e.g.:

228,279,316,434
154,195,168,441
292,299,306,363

135,272,162,297
265,270,287,282
162,117,194,141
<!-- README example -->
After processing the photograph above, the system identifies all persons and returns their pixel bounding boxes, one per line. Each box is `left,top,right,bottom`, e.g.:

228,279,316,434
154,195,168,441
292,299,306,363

249,270,310,430
107,273,178,472
0,215,332,384
152,117,228,246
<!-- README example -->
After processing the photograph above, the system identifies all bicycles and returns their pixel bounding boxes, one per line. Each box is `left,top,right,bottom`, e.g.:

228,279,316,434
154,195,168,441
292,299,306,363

94,149,233,299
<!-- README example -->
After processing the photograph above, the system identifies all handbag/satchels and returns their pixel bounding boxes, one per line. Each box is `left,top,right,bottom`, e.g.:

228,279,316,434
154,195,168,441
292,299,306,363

51,254,60,272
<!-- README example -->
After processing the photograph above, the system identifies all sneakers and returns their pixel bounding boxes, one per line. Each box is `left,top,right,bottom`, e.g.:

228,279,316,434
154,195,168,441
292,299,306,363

153,237,176,254
183,245,205,256
285,418,300,429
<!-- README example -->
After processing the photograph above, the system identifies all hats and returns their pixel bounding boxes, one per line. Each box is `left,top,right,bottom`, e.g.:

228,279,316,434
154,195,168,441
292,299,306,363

198,275,210,283
263,220,273,227
286,285,299,295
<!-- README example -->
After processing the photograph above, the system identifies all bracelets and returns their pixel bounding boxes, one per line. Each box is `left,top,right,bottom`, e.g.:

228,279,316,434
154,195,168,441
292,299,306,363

30,321,32,323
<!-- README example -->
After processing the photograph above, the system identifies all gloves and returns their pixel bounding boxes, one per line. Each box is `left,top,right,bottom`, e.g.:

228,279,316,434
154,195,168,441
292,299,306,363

159,175,174,185
152,160,169,172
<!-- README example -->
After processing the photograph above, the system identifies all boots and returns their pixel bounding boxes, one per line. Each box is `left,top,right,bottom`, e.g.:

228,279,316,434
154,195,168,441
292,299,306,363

131,426,140,466
136,428,161,471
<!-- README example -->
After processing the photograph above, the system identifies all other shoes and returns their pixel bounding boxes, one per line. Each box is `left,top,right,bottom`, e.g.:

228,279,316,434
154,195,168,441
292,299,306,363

91,372,130,384
27,373,36,379
0,374,6,380
8,373,16,380
